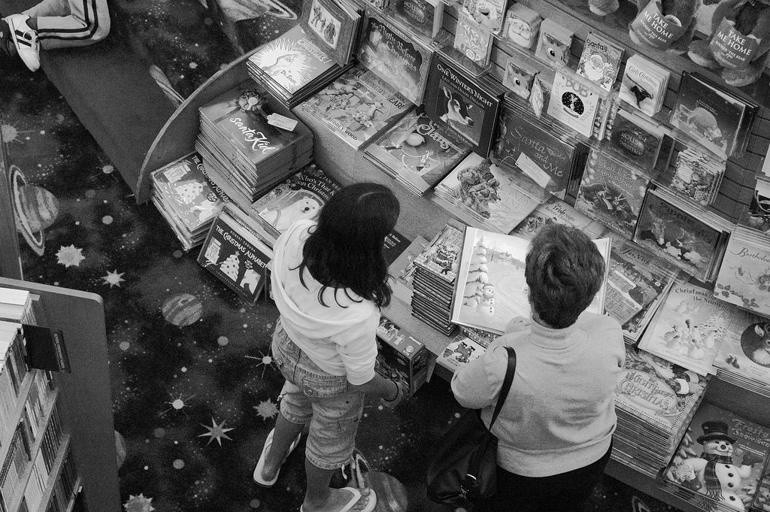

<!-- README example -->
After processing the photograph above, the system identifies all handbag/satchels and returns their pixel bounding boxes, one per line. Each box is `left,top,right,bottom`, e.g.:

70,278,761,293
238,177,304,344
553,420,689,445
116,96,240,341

424,408,500,510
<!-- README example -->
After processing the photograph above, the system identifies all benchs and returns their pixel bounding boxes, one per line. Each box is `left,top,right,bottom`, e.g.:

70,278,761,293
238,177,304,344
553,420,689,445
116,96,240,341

0,0,180,205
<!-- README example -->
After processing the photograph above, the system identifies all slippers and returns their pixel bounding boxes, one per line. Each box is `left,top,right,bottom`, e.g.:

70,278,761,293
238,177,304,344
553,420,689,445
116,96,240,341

253,426,301,488
300,487,377,512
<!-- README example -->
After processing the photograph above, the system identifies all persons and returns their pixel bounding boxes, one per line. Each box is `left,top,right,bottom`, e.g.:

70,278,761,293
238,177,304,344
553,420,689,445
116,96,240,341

0,0,112,72
451,223,625,512
254,183,402,512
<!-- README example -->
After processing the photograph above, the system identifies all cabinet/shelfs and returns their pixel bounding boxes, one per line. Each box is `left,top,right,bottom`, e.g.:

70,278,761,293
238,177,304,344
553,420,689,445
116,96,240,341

136,0,770,511
0,276,122,512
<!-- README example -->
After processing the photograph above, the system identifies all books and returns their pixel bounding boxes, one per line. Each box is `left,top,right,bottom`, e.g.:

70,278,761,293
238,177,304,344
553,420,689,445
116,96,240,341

153,1,547,398
547,30,770,511
0,287,84,512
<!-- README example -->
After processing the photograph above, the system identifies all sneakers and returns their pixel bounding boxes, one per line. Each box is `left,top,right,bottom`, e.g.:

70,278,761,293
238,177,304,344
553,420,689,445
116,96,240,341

0,14,41,73
